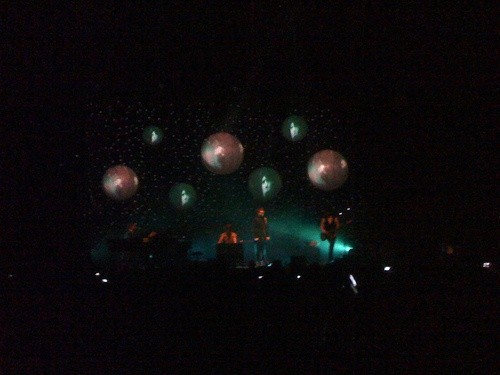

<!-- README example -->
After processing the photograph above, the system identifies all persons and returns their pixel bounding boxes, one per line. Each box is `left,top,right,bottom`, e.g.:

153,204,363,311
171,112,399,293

124,221,142,241
319,208,338,265
251,207,270,268
217,224,238,244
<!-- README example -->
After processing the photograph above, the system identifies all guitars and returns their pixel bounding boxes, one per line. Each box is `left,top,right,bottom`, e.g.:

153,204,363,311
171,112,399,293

320,218,353,242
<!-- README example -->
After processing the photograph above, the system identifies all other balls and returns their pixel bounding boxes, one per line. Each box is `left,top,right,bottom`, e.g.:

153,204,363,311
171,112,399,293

101,164,140,203
305,148,350,193
200,131,246,177
